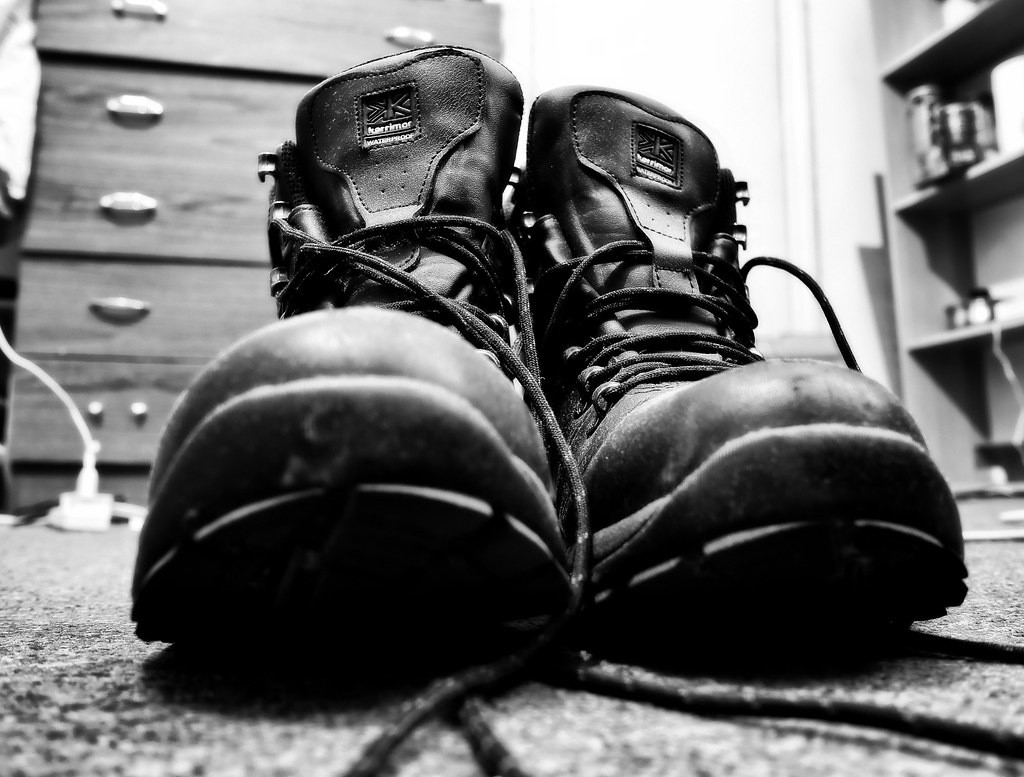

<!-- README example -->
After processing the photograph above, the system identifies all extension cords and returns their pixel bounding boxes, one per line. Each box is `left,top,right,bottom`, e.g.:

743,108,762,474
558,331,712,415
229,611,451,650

46,491,113,532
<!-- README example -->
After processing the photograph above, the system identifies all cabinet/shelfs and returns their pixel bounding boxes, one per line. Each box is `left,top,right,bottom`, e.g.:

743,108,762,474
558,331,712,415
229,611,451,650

8,0,499,517
866,2,1024,541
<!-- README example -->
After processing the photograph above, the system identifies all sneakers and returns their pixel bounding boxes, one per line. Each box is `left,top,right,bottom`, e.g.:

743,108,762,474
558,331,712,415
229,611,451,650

508,83,965,639
133,44,585,648
